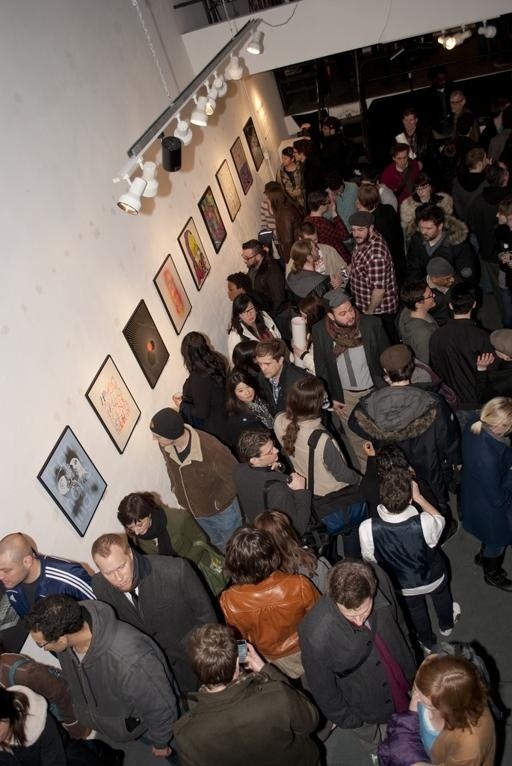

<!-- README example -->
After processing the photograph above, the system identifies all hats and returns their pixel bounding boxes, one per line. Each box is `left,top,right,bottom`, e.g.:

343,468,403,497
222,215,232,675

150,407,186,441
379,344,412,371
427,257,454,276
489,328,512,355
321,291,353,308
348,211,375,226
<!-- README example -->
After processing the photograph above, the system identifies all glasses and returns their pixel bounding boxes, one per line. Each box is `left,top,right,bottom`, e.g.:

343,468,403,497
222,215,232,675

35,639,51,650
416,185,429,192
240,252,259,260
240,306,255,317
293,151,301,155
323,200,331,205
422,290,436,302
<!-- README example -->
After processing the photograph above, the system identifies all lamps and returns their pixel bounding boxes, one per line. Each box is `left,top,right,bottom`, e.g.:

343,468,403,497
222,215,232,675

224,52,246,82
189,95,208,128
174,114,193,148
436,29,455,50
478,20,498,38
247,27,267,57
200,82,220,117
155,134,184,174
452,23,475,46
137,160,160,200
115,174,147,218
213,71,230,96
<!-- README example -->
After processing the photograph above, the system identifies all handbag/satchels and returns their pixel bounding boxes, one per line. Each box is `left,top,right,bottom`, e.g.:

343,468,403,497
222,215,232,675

9,659,67,723
413,363,460,410
262,479,338,563
304,428,381,536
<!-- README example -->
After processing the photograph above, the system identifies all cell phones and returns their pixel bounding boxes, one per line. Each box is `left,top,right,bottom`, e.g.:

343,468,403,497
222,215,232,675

236,640,248,664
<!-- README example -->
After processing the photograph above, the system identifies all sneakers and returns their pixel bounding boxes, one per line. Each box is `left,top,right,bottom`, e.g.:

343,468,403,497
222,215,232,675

440,602,461,636
484,573,512,592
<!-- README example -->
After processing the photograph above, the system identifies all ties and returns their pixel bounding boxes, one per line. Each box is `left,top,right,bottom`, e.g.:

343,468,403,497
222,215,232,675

129,589,140,609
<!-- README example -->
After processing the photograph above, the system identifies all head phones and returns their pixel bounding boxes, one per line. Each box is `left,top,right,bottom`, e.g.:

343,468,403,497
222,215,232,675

322,119,336,136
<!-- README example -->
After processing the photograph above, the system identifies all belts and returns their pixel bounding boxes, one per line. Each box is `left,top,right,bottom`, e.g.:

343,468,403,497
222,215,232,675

345,385,375,393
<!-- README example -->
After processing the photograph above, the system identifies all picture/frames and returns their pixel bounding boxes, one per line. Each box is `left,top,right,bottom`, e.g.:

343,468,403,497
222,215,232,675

123,299,171,390
151,253,196,335
216,159,242,222
243,116,264,171
176,217,213,293
36,424,108,539
198,185,228,253
85,353,141,454
230,137,254,196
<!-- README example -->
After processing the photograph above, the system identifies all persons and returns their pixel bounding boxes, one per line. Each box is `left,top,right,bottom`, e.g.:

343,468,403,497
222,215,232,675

428,70,460,116
218,528,325,680
260,190,278,247
359,169,399,213
274,146,313,210
340,212,401,314
496,200,512,225
448,90,483,143
470,161,512,321
241,239,285,307
480,97,508,145
379,143,424,212
227,272,253,301
284,222,347,293
258,511,339,595
170,623,323,766
313,288,396,476
461,396,512,592
452,148,495,223
357,475,462,653
304,191,354,265
272,376,365,563
452,109,483,158
423,112,466,184
1,532,97,621
399,172,454,261
149,407,239,556
488,105,512,158
255,339,312,413
173,331,235,454
0,683,126,766
479,204,512,328
27,595,178,766
356,186,405,288
183,230,208,285
225,371,279,440
403,206,486,309
396,285,441,365
429,284,493,412
371,443,438,514
320,175,364,238
473,329,512,402
283,239,336,307
392,638,504,766
314,117,352,153
346,344,462,547
232,342,262,378
91,535,218,697
224,296,284,360
265,181,309,261
296,563,421,729
394,109,432,161
292,139,342,206
232,426,316,534
288,296,320,376
420,256,466,328
116,491,233,596
